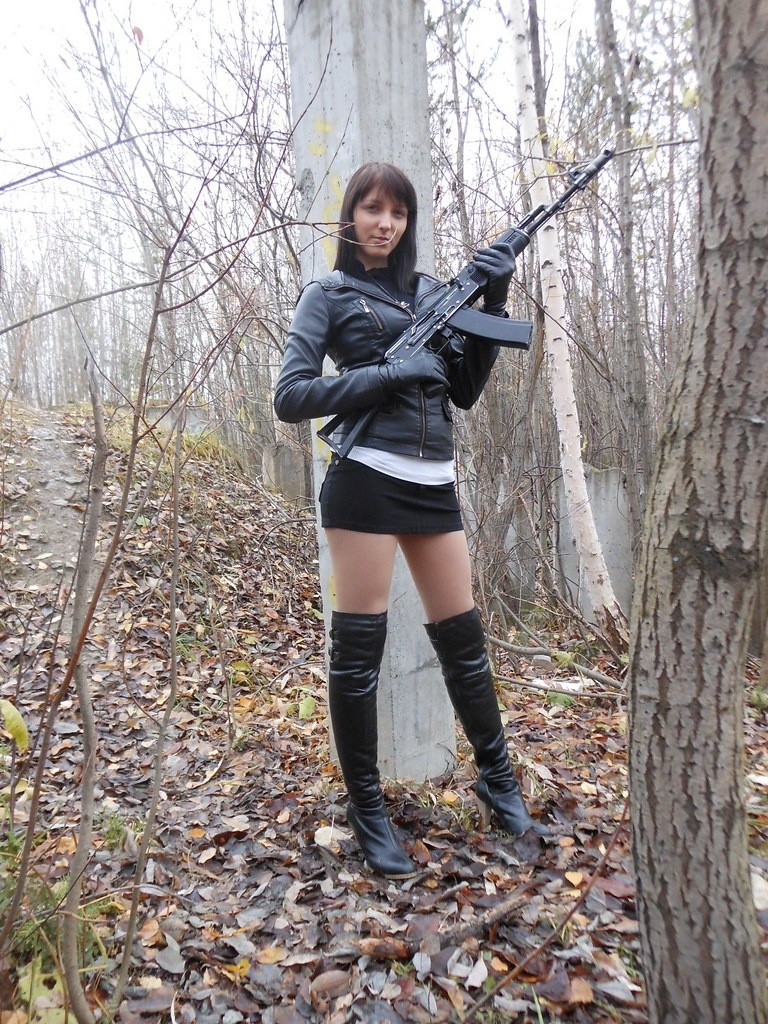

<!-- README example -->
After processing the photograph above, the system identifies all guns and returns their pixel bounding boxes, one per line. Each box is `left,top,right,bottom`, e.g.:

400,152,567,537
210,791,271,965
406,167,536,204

315,148,616,463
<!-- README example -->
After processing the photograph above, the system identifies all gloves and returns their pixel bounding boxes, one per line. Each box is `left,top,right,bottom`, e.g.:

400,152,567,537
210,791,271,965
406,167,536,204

379,353,451,389
473,243,516,318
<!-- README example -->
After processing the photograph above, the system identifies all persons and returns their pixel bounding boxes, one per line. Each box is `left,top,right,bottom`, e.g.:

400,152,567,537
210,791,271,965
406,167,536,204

274,161,551,880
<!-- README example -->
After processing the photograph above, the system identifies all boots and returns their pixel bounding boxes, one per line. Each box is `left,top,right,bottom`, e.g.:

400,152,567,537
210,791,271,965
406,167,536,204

328,611,415,875
422,603,554,839
